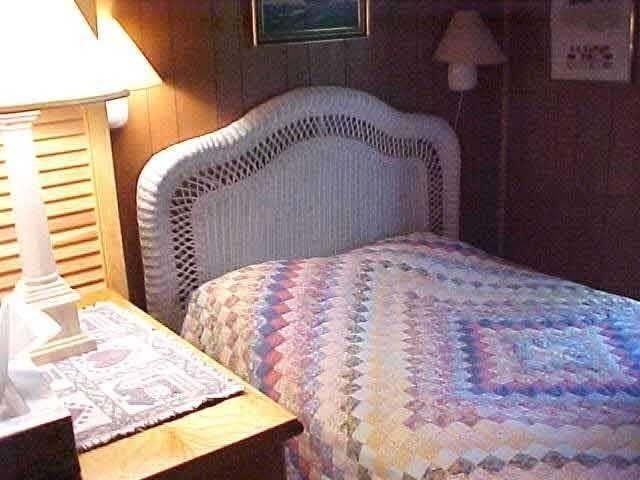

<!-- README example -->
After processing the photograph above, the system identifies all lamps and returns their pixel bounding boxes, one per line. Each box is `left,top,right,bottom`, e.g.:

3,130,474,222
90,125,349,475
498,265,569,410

430,11,507,92
2,0,129,369
97,15,161,129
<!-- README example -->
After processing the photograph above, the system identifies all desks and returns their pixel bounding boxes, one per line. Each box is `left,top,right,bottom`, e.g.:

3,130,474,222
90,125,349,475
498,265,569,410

0,291,302,479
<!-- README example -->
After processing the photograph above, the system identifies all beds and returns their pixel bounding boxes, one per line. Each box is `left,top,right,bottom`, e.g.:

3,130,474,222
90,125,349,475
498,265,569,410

136,87,640,480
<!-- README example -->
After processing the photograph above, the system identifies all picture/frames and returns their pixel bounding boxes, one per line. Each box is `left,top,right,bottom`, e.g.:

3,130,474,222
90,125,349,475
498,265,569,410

251,0,370,48
